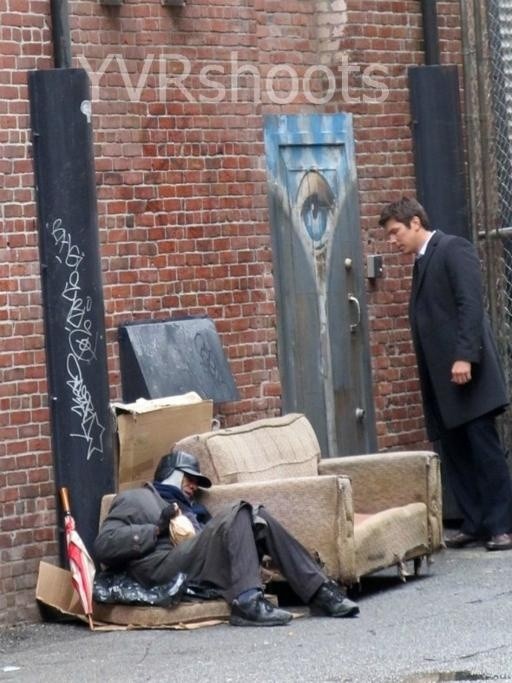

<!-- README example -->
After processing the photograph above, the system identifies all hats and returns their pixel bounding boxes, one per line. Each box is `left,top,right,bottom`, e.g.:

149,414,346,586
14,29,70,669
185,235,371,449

155,452,211,488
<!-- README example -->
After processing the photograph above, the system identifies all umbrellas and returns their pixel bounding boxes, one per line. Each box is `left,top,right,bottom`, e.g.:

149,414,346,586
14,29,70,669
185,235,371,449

58,487,97,632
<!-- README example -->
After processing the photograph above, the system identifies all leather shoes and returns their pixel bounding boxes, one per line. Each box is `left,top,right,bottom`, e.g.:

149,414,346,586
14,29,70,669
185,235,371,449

486,531,512,549
444,530,487,547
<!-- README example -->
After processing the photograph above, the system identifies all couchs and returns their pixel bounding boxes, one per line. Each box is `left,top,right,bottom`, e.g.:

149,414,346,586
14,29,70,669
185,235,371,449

176,414,447,595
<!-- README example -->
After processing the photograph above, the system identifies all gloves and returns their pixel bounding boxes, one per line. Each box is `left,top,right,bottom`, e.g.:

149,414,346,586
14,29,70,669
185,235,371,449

157,502,180,536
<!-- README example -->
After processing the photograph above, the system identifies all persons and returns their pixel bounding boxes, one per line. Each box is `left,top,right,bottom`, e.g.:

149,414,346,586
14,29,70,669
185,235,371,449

93,447,358,626
378,194,512,549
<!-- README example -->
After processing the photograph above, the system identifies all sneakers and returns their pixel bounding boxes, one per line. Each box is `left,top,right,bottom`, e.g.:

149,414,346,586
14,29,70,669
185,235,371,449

310,583,359,616
230,591,294,626
